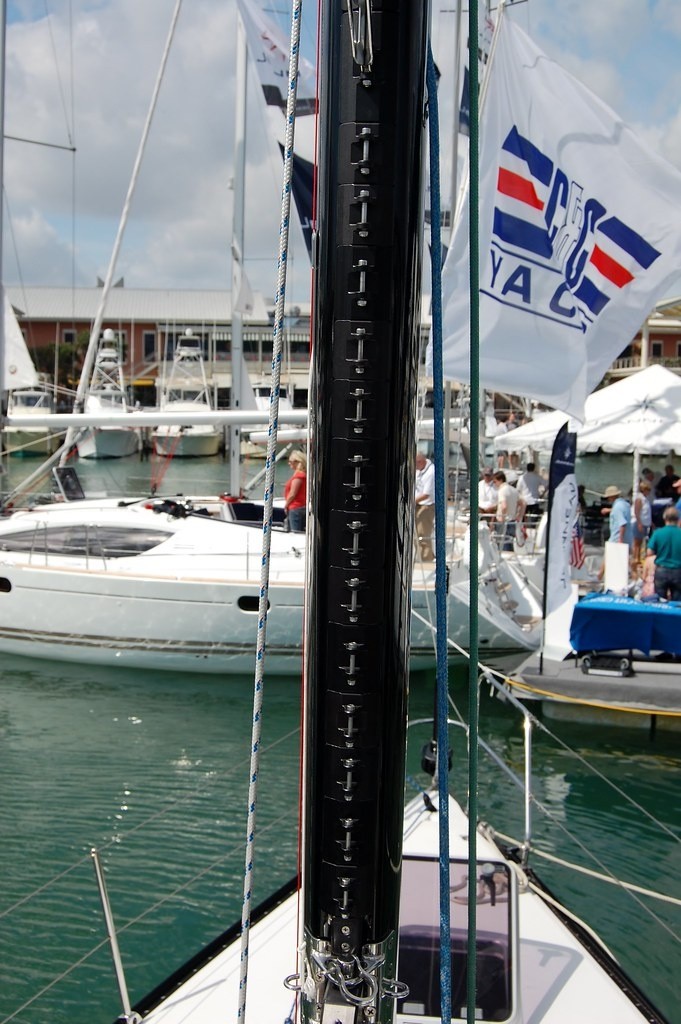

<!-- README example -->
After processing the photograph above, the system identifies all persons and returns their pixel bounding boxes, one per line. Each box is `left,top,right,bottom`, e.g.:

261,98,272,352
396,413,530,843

515,462,549,529
647,507,681,601
577,484,587,517
477,467,526,553
640,464,681,528
588,486,632,581
415,450,438,561
630,482,651,562
496,411,532,469
284,449,307,531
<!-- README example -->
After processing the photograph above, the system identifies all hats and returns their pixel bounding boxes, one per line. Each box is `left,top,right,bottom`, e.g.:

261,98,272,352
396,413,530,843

483,467,493,475
603,486,622,498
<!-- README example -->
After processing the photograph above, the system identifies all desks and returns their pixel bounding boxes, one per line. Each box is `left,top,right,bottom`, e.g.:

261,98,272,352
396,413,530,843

570,591,681,677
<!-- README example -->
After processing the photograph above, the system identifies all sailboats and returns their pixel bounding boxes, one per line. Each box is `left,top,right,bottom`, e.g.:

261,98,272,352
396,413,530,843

0,0,681,1024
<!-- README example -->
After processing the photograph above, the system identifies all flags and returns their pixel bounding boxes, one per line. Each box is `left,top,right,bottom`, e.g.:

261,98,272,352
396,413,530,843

238,0,318,265
428,11,681,423
570,519,587,569
